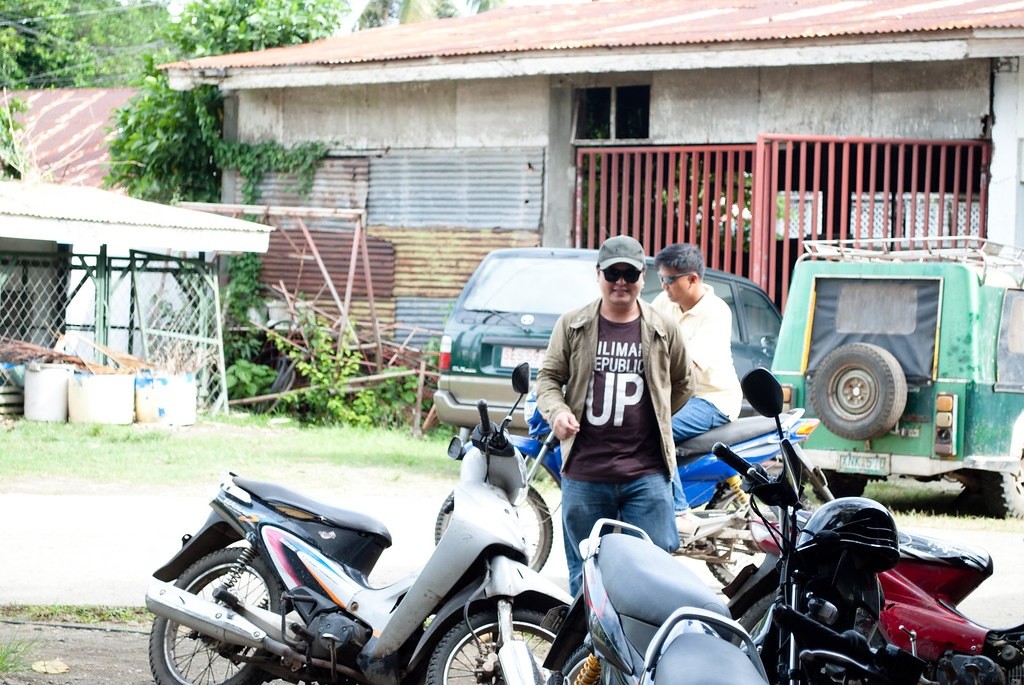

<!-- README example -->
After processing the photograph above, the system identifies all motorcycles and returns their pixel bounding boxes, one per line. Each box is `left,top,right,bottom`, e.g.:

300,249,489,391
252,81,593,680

144,364,1024,684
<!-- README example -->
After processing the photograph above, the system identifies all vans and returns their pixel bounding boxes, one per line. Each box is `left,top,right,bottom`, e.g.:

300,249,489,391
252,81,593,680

432,247,783,441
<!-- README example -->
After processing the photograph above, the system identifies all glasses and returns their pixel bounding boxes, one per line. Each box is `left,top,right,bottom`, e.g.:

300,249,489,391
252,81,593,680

600,266,641,284
656,273,700,285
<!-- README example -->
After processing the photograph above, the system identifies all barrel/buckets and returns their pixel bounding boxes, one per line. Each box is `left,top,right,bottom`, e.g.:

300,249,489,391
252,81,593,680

25,363,71,421
69,374,135,425
136,370,198,425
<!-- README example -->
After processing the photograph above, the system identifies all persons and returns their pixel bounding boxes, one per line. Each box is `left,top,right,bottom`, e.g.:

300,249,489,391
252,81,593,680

650,243,744,517
535,234,693,599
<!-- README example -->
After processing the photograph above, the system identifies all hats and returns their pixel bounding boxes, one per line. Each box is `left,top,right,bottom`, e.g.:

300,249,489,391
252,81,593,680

597,235,645,273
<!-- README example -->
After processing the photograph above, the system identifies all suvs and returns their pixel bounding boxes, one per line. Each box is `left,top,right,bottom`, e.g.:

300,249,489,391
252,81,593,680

766,229,1024,519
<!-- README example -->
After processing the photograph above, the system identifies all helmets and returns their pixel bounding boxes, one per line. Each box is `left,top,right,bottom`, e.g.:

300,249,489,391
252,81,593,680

796,497,900,573
